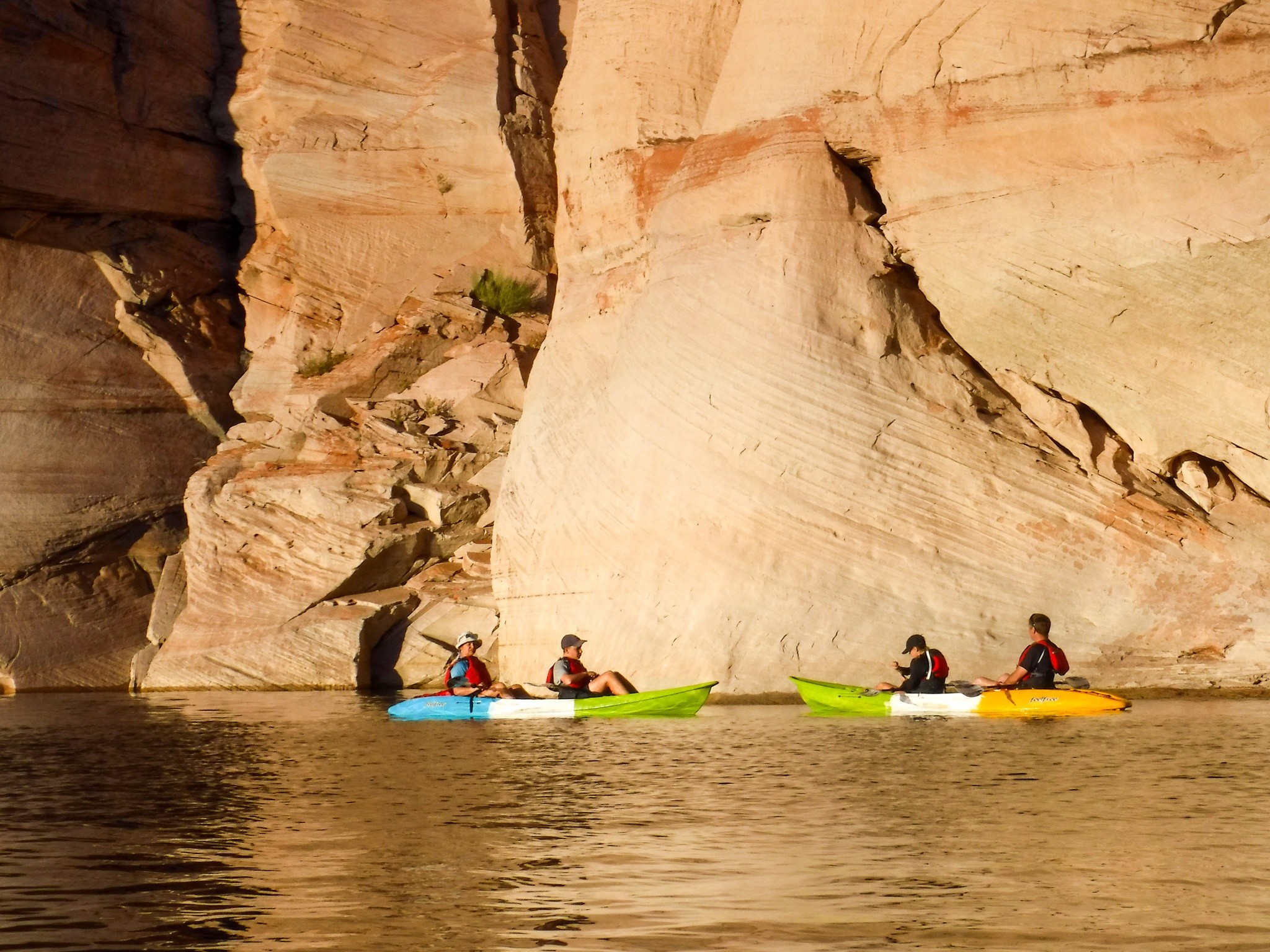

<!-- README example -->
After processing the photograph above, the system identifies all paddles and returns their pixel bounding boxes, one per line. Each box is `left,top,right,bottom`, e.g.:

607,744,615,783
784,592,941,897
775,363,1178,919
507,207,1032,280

522,682,614,696
954,676,1090,698
853,680,971,697
446,675,484,690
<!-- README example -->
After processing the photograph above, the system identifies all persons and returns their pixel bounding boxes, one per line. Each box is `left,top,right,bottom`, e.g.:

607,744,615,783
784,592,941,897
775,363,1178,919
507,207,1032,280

552,634,640,699
972,612,1056,690
445,632,536,700
875,634,949,695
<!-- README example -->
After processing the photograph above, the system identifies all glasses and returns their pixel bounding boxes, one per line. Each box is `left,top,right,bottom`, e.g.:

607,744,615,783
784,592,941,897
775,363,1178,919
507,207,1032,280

459,634,478,642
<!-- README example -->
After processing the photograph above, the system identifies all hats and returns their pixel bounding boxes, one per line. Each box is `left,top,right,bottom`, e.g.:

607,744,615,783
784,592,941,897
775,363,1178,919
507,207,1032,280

455,631,482,648
561,635,587,649
902,635,927,654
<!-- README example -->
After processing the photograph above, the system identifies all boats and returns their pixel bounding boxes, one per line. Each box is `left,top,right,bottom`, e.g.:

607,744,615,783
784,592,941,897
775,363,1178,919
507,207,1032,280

786,675,1133,719
387,680,719,721
